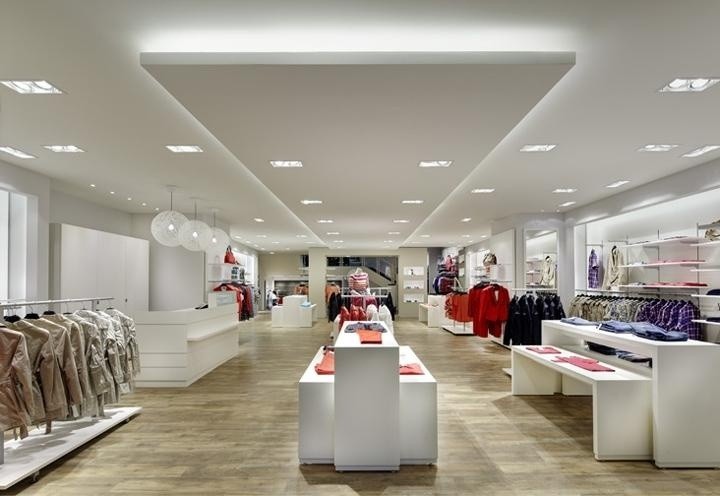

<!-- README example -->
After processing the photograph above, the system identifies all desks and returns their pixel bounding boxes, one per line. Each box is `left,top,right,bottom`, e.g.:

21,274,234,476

510,343,656,462
539,317,720,470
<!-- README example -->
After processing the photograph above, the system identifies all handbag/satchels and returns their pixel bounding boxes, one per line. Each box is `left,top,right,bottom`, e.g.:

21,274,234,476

225,246,236,263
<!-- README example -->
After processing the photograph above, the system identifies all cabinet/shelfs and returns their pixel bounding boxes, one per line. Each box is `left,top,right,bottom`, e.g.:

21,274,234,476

474,263,500,282
299,321,438,472
211,263,246,282
271,294,317,327
615,234,709,291
526,260,542,287
339,287,391,305
419,294,450,328
688,220,720,325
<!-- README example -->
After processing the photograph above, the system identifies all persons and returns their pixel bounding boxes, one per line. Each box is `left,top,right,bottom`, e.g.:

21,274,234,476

347,267,371,296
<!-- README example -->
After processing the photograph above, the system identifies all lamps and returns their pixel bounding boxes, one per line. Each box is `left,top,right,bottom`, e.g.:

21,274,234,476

180,194,214,252
199,206,231,257
151,184,191,248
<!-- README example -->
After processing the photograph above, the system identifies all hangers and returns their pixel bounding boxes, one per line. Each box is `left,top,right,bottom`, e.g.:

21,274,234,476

590,247,595,254
340,296,385,314
0,295,116,323
545,255,549,259
217,280,253,294
448,281,562,303
612,242,617,251
573,289,694,317
